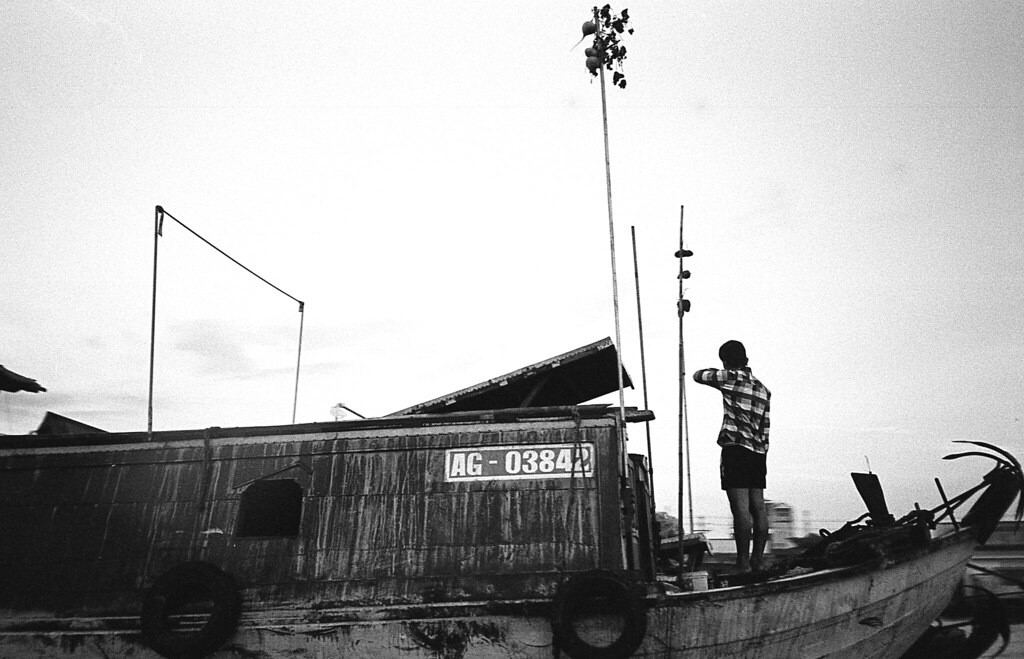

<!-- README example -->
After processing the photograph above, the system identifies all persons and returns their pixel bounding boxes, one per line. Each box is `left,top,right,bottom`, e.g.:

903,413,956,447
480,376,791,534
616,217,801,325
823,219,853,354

692,339,771,575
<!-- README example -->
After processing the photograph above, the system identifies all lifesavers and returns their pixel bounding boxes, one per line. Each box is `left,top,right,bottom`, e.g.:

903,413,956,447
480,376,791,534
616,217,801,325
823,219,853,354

551,567,650,659
140,560,244,659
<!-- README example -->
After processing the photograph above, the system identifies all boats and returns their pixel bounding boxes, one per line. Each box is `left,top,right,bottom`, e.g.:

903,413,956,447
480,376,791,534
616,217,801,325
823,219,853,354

0,401,1023,659
